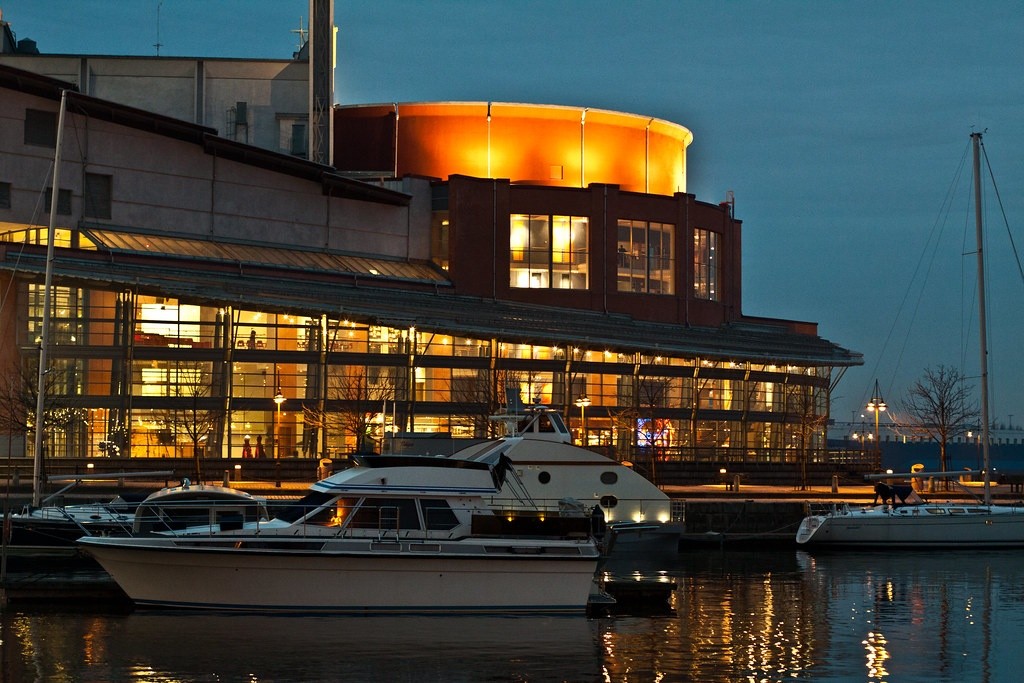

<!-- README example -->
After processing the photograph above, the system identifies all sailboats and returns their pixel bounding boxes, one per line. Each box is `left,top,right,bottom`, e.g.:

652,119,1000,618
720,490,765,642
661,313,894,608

0,88,269,546
796,132,1023,546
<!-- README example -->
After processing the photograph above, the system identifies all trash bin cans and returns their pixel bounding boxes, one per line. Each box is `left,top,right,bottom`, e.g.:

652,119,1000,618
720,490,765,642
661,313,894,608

621,460,633,470
319,458,333,480
910,463,924,492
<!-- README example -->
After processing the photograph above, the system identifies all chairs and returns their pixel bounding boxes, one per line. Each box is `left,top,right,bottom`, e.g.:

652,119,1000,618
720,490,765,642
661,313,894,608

297,342,309,351
237,340,264,350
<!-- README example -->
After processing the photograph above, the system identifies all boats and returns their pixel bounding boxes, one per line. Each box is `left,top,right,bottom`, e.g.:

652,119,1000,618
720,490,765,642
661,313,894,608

73,453,617,617
957,482,1024,495
446,399,686,554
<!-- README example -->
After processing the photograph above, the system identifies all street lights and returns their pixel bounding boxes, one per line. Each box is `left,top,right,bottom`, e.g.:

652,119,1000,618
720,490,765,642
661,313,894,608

865,378,886,474
273,392,287,488
967,417,983,470
852,421,874,450
574,374,591,449
